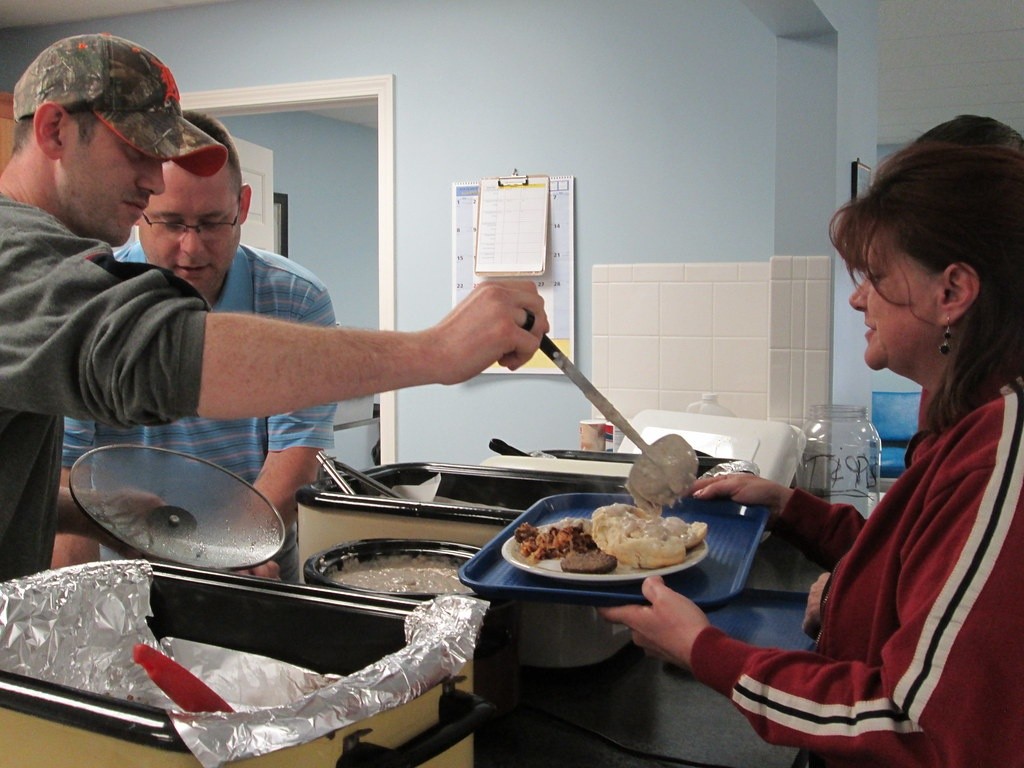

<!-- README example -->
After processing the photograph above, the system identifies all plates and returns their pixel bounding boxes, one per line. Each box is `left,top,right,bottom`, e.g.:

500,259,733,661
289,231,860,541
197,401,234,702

501,522,709,582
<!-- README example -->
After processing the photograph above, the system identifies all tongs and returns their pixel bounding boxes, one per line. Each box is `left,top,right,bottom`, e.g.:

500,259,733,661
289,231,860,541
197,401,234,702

315,450,403,499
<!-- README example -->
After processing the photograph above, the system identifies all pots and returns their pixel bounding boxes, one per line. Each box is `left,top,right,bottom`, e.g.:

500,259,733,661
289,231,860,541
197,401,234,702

304,538,482,599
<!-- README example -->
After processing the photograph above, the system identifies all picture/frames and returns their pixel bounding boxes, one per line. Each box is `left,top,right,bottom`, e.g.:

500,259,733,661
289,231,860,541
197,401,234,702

273,192,289,258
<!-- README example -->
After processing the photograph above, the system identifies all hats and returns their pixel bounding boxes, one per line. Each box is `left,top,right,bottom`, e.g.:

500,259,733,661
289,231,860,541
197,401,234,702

12,33,227,177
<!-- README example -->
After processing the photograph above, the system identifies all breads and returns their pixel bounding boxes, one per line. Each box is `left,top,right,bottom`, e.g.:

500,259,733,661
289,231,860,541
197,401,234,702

592,503,708,569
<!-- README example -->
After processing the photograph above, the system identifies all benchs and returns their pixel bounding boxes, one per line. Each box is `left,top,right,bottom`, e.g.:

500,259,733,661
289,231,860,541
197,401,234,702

868,390,921,493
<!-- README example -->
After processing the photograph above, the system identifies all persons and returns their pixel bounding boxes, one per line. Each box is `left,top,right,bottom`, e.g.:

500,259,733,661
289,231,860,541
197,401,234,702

48,109,338,581
597,115,1024,768
0,34,551,583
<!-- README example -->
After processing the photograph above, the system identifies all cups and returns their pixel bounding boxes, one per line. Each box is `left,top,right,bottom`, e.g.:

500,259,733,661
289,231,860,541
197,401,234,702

579,419,614,453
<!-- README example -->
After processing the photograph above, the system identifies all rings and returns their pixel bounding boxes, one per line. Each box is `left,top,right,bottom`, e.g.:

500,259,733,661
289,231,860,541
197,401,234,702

520,306,536,330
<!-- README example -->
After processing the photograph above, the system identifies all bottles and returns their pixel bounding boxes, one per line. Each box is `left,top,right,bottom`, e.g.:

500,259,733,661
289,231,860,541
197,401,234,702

795,404,883,520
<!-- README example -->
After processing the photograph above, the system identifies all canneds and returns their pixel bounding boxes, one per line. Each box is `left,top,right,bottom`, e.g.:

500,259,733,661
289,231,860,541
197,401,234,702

578,419,614,452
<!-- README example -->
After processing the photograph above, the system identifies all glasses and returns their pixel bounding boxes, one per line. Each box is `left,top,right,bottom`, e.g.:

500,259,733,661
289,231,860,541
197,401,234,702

142,190,243,242
